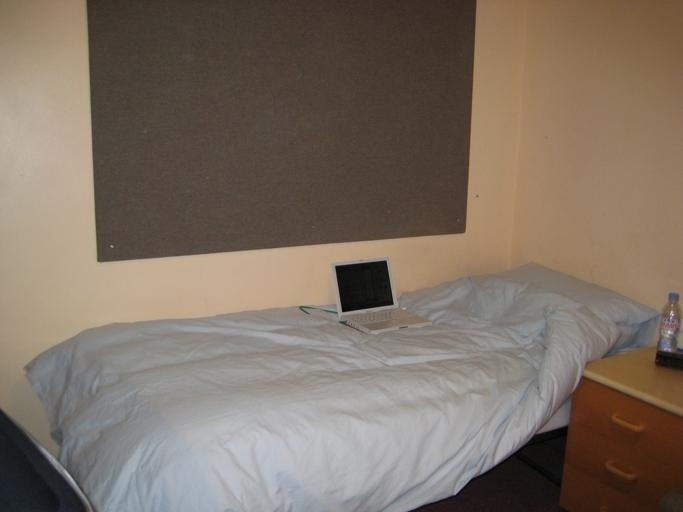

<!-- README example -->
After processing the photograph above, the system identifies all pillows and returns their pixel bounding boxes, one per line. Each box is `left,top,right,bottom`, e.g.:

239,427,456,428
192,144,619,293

502,260,659,353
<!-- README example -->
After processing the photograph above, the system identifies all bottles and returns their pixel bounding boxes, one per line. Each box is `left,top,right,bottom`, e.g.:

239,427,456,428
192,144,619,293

658,292,680,354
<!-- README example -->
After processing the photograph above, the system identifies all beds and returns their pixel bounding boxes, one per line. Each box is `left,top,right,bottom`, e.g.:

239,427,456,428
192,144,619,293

22,274,666,511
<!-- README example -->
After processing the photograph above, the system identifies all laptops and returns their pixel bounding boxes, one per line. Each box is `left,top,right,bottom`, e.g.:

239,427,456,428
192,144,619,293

330,256,433,335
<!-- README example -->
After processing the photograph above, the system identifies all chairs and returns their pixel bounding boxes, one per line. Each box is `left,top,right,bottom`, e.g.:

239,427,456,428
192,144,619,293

0,406,94,511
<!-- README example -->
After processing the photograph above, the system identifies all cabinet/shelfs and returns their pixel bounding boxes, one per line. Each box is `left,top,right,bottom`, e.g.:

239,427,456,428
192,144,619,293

557,347,682,512
